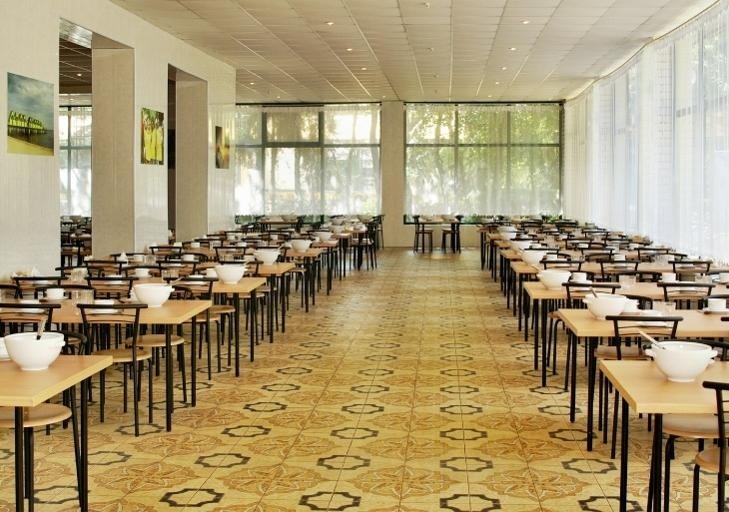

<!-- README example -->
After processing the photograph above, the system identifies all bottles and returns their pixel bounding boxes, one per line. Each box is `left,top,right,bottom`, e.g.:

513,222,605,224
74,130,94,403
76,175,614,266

467,214,494,222
542,211,556,222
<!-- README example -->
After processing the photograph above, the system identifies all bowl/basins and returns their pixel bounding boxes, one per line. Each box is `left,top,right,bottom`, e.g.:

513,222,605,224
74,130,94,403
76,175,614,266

651,341,713,384
216,265,244,284
84,233,246,280
6,332,64,372
497,225,572,290
423,213,455,222
587,294,628,322
280,214,298,222
134,282,172,308
256,217,363,266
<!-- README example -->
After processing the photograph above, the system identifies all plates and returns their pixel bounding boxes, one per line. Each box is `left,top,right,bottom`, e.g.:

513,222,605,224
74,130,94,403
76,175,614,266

91,309,119,314
43,297,68,303
20,299,46,315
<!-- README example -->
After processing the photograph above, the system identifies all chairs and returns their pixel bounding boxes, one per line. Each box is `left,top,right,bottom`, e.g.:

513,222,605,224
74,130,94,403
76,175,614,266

414,214,462,255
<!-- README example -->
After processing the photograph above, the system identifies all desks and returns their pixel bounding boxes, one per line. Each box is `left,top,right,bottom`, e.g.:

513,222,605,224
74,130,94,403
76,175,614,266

402,212,479,252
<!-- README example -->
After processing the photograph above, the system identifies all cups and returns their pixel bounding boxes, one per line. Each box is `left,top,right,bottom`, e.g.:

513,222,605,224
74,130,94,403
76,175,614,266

47,289,63,299
573,251,729,313
95,300,114,305
